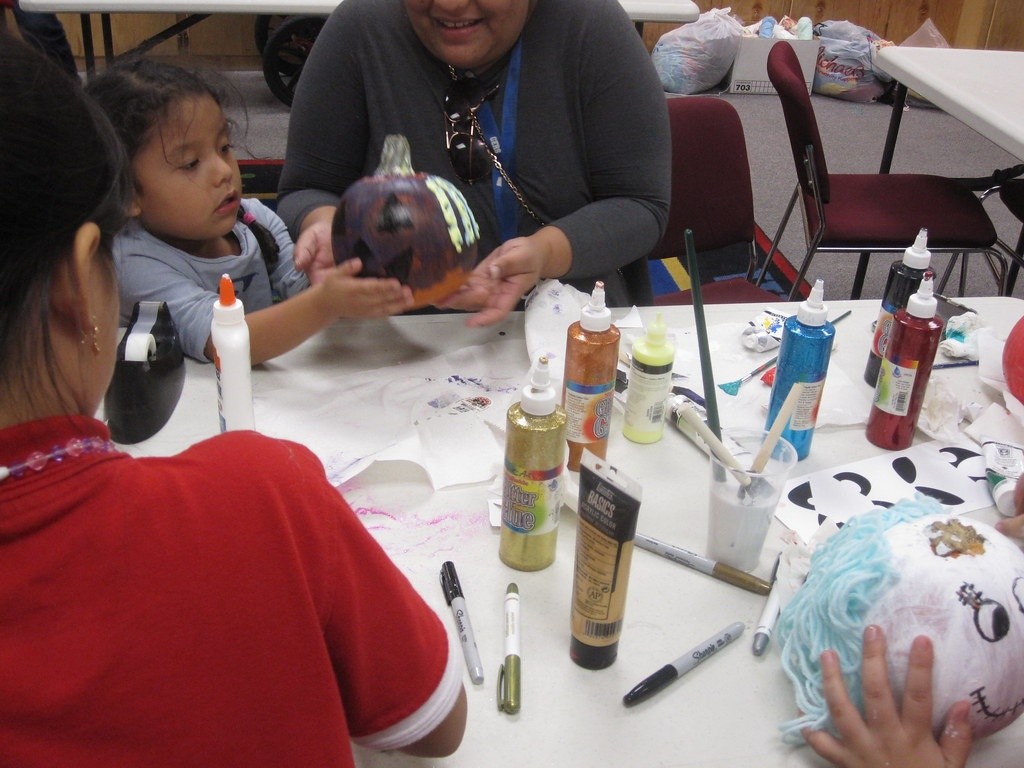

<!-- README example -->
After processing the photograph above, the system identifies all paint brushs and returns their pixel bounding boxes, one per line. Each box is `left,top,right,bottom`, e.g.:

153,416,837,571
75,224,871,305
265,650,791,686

717,311,853,396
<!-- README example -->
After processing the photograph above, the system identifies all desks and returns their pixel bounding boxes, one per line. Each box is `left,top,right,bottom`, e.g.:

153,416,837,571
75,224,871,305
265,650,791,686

877,47,1024,301
618,1,701,37
100,297,1024,767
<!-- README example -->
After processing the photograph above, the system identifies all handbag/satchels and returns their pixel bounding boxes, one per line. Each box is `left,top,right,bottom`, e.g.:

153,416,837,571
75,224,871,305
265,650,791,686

811,19,896,103
650,7,742,95
718,20,821,95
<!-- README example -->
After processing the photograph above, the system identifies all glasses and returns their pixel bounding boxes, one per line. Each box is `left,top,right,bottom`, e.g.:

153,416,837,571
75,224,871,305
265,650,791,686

441,69,498,185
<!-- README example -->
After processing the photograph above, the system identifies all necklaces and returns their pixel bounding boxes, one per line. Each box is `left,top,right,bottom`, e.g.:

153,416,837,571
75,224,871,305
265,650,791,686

0,436,118,480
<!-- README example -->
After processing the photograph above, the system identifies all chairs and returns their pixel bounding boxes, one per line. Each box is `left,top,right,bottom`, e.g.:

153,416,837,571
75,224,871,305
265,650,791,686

767,41,1008,304
644,97,811,303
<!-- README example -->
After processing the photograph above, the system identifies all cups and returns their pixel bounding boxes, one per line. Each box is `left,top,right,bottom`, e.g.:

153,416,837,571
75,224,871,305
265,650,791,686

706,428,798,575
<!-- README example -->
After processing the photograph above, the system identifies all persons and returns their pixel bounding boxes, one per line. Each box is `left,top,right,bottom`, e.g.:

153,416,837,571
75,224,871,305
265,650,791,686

0,31,469,768
798,472,1024,768
276,0,673,329
82,58,413,366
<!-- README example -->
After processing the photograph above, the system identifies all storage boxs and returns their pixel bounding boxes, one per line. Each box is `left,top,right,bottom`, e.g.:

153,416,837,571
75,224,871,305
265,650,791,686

729,39,820,95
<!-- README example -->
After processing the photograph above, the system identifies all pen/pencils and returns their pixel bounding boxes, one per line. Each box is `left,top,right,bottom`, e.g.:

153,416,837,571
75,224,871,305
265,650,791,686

496,583,521,715
439,561,483,685
621,623,743,708
933,360,979,369
633,535,772,594
752,553,790,657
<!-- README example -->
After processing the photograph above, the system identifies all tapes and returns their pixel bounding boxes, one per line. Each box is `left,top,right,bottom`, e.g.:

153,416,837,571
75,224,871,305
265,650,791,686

125,333,157,361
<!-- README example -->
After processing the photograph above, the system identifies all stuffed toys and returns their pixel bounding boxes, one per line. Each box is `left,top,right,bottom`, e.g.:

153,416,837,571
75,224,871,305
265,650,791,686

781,491,1024,749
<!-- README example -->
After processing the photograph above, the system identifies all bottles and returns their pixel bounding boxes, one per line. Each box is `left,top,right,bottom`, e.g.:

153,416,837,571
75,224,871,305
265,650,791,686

622,312,674,444
498,357,567,573
561,281,621,472
211,274,254,436
763,278,836,464
863,227,931,388
865,271,945,451
